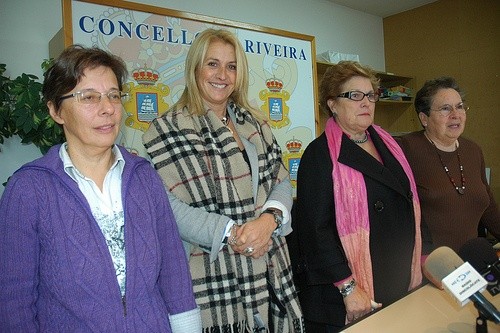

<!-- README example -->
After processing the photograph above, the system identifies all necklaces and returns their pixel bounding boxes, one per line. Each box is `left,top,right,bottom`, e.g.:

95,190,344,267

222,110,235,134
352,135,366,144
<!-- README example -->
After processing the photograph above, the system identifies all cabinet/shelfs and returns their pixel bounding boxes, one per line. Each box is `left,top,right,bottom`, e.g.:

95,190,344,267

317,60,416,134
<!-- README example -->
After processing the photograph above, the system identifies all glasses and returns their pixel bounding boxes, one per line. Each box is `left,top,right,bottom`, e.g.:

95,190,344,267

337,91,381,103
433,104,470,116
56,88,130,104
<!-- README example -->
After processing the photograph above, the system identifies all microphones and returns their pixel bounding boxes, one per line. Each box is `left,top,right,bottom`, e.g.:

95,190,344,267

459,238,500,297
425,246,500,326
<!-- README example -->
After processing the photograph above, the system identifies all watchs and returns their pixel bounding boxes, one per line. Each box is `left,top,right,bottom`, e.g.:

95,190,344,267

258,209,283,230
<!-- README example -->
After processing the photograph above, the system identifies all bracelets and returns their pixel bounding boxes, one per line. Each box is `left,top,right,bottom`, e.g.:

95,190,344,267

338,279,356,296
230,224,236,243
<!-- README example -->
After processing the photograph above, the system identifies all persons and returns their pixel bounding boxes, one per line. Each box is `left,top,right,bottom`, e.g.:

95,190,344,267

142,30,306,333
276,60,423,333
0,44,203,333
398,78,500,253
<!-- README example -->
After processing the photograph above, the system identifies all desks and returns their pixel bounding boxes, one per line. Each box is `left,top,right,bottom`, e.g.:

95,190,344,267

340,241,500,333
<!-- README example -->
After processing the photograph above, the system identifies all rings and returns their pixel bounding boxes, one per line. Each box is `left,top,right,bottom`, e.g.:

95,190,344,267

371,301,377,309
425,130,466,195
354,315,358,320
245,248,254,253
